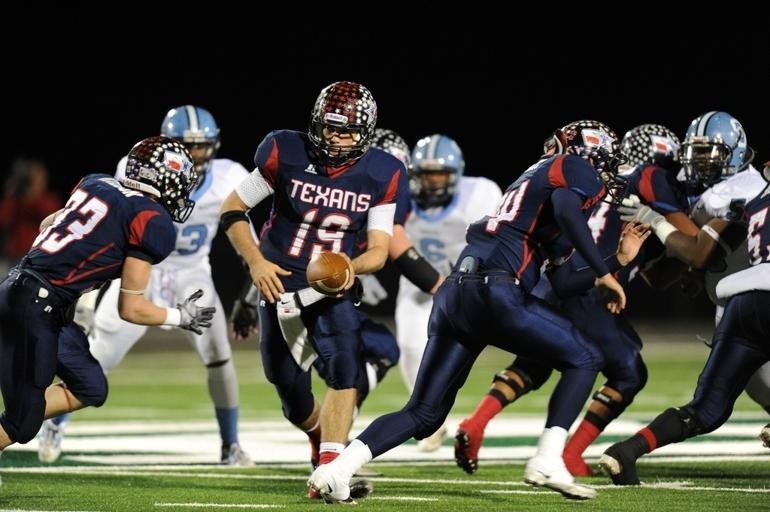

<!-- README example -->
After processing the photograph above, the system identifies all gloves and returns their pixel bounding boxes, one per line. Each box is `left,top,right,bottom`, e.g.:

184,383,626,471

165,289,215,335
617,202,676,242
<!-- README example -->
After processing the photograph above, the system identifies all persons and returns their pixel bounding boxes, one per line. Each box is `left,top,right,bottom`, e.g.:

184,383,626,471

0,156,61,282
348,128,446,446
599,161,770,486
453,123,700,478
395,135,503,451
0,137,218,459
38,106,259,467
616,112,770,420
308,120,650,505
219,80,408,499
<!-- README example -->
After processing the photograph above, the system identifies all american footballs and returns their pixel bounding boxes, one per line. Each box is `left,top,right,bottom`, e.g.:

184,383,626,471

306,252,351,295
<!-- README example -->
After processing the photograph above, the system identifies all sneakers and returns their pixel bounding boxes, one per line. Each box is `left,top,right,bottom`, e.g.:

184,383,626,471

221,442,255,468
761,424,770,448
456,418,483,473
523,451,596,500
416,416,461,453
600,442,641,486
306,467,374,506
562,447,594,477
39,417,65,463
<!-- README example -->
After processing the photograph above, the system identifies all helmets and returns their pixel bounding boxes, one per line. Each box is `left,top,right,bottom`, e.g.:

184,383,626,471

677,111,756,186
162,105,220,183
370,129,410,172
126,137,197,222
620,123,683,175
553,119,629,208
307,81,377,166
412,137,465,206
